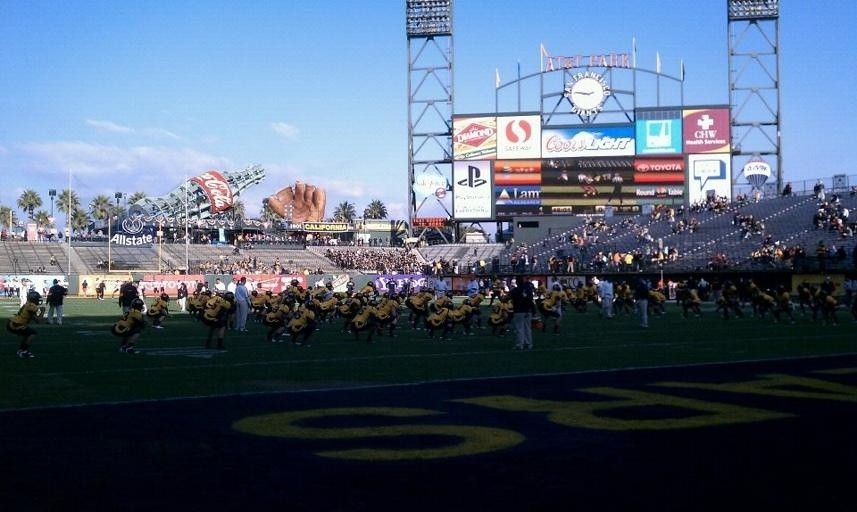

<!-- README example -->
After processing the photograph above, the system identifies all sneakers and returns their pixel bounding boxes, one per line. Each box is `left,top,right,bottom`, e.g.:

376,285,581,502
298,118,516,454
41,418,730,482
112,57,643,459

16,347,40,359
119,343,140,356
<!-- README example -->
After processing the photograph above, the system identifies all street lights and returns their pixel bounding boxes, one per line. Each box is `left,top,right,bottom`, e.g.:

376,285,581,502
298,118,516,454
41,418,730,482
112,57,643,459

283,205,293,225
49,189,56,217
115,192,121,234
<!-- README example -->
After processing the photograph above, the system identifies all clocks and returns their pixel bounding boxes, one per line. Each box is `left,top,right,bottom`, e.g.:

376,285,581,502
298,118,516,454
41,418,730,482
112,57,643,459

570,77,604,111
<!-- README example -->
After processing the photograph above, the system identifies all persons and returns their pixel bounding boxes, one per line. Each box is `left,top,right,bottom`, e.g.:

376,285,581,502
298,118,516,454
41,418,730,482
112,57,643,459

71,232,88,241
198,255,324,275
305,233,382,247
510,178,857,274
675,275,841,331
37,267,46,273
248,278,486,346
19,278,29,308
486,278,666,350
173,232,177,244
2,279,20,298
109,280,171,357
65,230,69,243
51,255,55,266
233,247,240,256
324,248,459,278
81,280,88,297
45,279,68,325
238,233,301,245
177,278,250,353
6,292,45,359
97,259,115,270
95,278,106,301
38,229,51,243
58,231,63,242
473,247,477,256
201,235,208,244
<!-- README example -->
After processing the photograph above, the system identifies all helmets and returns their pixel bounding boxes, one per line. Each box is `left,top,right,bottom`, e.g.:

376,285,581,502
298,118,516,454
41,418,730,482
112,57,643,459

27,291,41,305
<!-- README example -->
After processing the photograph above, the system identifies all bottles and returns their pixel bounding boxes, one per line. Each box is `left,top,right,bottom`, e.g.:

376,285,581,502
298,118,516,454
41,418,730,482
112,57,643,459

127,164,266,221
